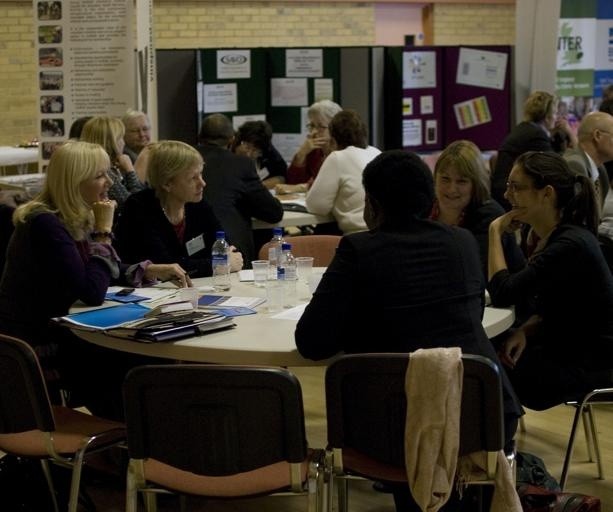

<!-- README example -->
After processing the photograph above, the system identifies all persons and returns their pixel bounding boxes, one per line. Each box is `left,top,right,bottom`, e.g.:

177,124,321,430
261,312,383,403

81,116,146,242
231,120,288,191
68,116,95,141
555,101,569,119
0,141,194,423
578,96,594,116
122,110,152,166
274,101,345,195
487,150,613,418
562,111,613,235
551,121,578,151
600,98,613,115
295,149,527,512
490,89,558,210
191,114,284,270
111,140,244,282
305,109,385,236
426,139,508,252
604,83,611,97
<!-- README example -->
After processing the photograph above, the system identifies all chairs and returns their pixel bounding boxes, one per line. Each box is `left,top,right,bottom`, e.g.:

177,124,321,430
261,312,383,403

260,235,347,268
124,363,321,512
324,355,515,511
1,334,123,511
560,388,612,486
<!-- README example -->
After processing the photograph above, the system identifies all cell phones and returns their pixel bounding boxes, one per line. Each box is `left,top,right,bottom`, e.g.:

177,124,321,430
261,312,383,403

115,288,134,297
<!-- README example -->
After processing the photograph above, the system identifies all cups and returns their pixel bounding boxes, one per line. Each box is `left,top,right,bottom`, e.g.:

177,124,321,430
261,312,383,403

251,260,269,286
294,256,313,282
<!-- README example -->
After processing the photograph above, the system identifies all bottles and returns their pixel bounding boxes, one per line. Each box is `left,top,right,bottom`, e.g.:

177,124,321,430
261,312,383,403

276,243,297,309
212,231,231,291
268,228,287,281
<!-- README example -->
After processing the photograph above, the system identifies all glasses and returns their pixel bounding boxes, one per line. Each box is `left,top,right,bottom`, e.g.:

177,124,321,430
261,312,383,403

507,180,537,193
307,124,330,132
241,143,262,156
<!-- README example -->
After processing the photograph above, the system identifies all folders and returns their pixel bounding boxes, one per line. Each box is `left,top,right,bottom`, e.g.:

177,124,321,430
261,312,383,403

59,302,154,334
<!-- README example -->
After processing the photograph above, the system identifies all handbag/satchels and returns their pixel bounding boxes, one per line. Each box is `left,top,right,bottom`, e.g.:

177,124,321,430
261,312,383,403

512,453,602,511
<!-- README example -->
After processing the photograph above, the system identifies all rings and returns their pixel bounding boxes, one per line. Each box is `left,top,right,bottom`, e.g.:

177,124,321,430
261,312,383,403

103,199,112,204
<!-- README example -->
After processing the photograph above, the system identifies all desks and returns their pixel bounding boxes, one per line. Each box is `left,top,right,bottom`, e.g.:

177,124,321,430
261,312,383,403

71,267,519,452
252,184,339,230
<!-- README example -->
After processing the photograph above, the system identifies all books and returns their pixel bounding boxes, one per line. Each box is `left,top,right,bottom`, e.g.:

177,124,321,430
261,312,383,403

58,299,153,332
